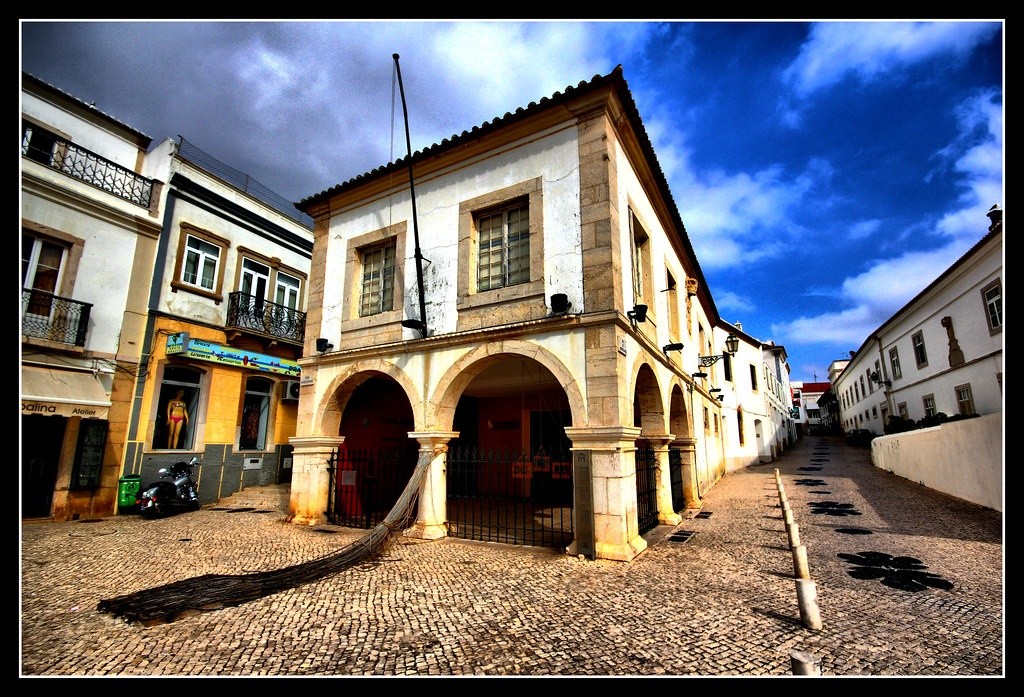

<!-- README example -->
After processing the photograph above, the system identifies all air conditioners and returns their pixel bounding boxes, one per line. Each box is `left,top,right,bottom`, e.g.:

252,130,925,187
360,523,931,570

281,380,300,401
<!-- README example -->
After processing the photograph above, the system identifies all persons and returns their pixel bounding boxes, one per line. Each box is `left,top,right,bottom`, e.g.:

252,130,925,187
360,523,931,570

165,390,188,450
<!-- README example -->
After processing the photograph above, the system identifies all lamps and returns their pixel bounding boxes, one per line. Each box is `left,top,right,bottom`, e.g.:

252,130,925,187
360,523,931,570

870,371,891,387
698,332,739,367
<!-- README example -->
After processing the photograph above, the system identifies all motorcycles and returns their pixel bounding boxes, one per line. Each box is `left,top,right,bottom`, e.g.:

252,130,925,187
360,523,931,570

135,455,200,519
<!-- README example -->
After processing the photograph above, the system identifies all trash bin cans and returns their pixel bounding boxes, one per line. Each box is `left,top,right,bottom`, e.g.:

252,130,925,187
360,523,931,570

118,474,143,507
